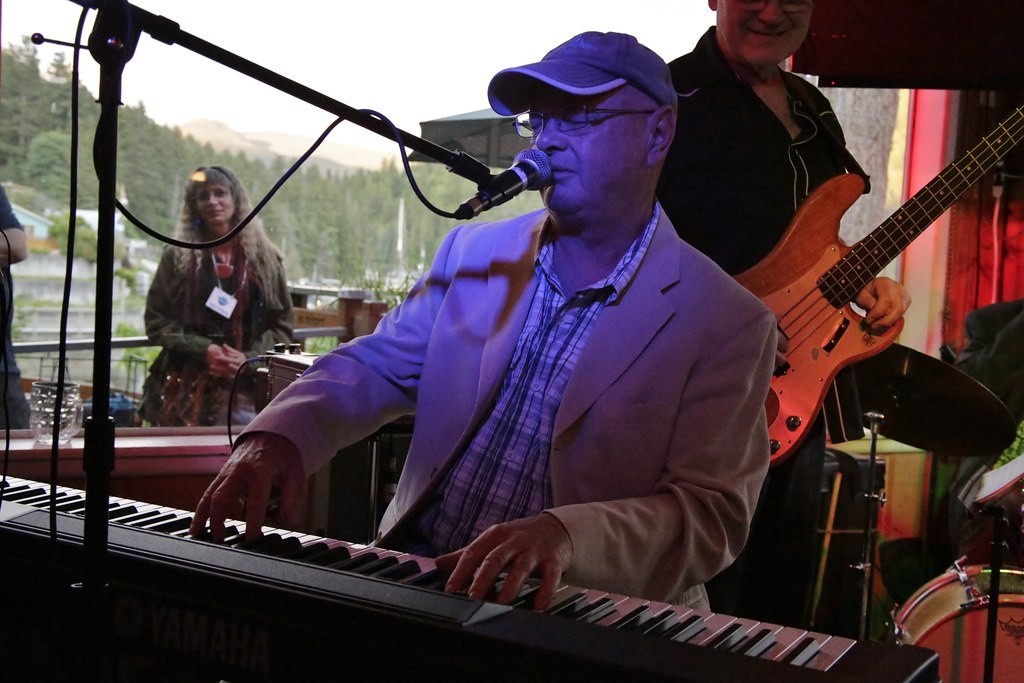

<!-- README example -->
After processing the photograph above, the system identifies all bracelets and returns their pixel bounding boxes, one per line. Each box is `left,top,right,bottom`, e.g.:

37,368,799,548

0,229,12,267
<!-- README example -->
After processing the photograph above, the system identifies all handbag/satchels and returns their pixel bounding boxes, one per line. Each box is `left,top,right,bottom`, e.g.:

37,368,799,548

154,371,210,427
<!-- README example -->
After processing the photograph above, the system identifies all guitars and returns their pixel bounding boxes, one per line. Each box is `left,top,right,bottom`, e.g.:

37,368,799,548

729,102,1023,481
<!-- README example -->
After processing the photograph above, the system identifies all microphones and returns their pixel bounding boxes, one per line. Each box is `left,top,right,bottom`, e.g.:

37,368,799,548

456,148,553,220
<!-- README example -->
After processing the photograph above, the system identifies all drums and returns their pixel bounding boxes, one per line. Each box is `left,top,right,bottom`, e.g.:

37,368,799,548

889,553,1023,683
953,455,1024,568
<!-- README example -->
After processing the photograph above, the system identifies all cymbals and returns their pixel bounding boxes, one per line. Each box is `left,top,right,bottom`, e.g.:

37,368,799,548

830,335,1018,456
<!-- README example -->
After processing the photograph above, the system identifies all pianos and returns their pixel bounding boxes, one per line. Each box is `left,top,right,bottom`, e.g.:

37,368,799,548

0,471,942,683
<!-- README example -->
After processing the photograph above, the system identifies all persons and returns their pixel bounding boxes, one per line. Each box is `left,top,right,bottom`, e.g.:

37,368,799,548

662,1,915,628
188,27,778,614
0,179,33,431
949,296,1024,565
134,165,296,431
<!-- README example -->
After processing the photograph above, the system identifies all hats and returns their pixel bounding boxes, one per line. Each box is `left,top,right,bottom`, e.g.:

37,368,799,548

487,30,678,116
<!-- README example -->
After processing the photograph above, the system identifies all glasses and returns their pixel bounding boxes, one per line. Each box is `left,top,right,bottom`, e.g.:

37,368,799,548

720,0,812,12
512,102,656,137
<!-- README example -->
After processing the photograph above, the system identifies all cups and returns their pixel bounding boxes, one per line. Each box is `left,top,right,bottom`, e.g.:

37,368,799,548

28,381,84,446
